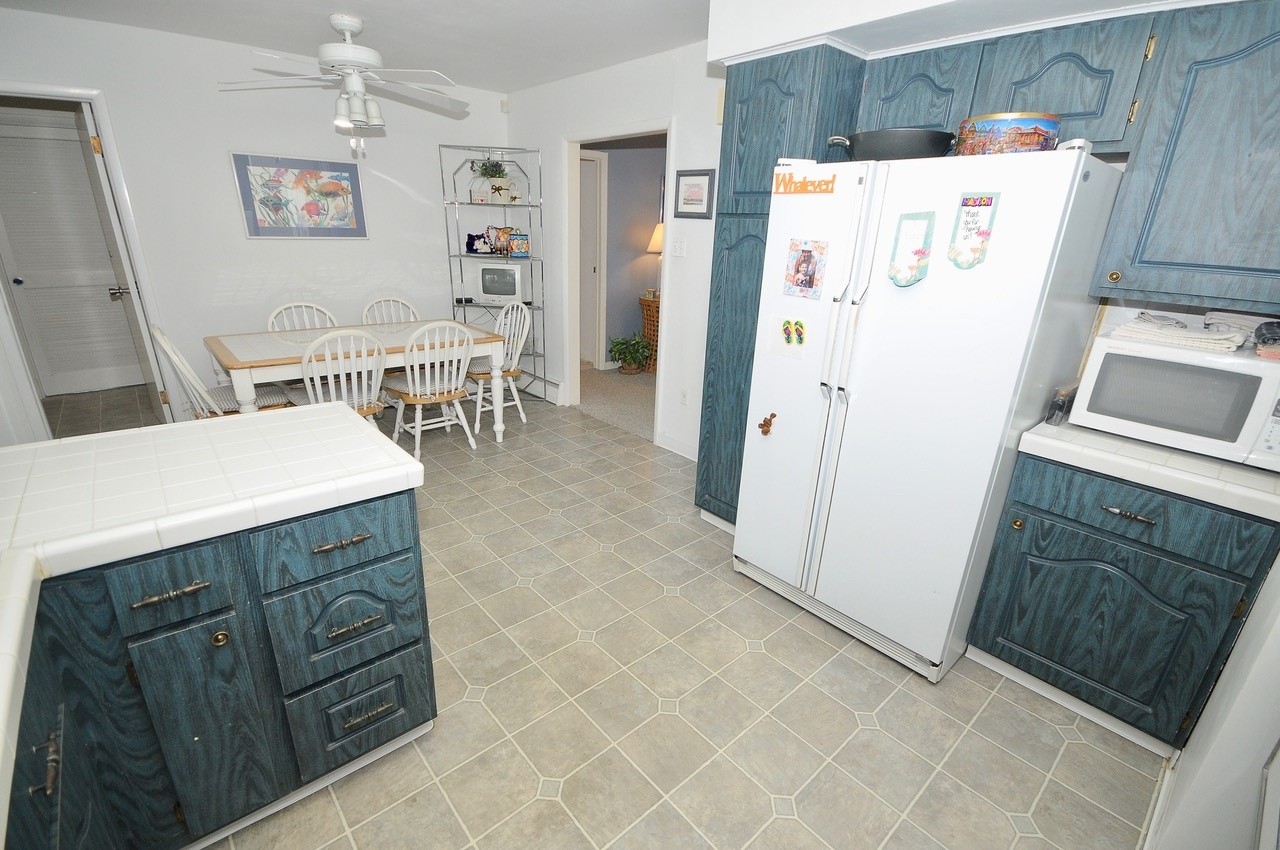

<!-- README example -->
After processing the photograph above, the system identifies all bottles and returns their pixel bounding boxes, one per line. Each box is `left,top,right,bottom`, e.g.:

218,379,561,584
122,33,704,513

646,288,656,298
509,232,529,257
1045,388,1068,426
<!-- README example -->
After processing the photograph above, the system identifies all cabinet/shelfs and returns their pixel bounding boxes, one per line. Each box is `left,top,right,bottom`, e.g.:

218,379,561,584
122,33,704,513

1088,0,1280,319
3,488,442,850
856,11,1172,163
694,34,867,537
438,143,547,402
963,447,1280,770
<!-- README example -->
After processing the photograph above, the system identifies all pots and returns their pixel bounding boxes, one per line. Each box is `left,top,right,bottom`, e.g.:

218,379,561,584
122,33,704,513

828,128,956,161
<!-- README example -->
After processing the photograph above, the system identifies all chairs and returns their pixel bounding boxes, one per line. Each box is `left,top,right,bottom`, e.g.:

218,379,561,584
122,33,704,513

151,297,533,463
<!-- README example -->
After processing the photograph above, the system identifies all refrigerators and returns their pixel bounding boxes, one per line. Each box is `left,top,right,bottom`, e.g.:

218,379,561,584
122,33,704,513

732,148,1123,683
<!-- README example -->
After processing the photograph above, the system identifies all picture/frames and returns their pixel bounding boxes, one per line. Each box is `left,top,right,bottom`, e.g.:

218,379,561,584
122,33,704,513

674,168,715,220
229,151,370,240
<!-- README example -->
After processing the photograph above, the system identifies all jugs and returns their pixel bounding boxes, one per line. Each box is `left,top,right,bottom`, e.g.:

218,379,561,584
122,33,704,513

486,225,513,255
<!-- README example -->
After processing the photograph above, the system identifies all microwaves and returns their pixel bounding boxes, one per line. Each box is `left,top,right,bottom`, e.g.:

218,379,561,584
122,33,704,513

1067,329,1280,473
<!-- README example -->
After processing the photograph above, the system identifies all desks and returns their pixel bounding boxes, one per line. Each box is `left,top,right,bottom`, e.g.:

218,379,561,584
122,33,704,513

204,319,506,444
638,296,661,375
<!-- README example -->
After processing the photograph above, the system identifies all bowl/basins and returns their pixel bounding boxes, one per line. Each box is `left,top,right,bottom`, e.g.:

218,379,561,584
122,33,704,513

955,113,1060,155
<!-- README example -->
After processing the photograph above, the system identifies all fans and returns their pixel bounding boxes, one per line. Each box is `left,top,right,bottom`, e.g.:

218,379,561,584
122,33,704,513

217,14,470,115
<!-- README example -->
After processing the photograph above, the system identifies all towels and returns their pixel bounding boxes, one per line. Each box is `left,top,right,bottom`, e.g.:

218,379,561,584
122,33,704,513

1109,310,1280,360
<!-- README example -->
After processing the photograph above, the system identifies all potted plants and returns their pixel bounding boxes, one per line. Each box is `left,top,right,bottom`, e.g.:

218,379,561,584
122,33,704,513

607,328,651,376
468,146,518,204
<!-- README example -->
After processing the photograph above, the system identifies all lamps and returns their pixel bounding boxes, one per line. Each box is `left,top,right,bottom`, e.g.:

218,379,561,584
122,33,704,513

645,223,664,297
333,92,386,151
